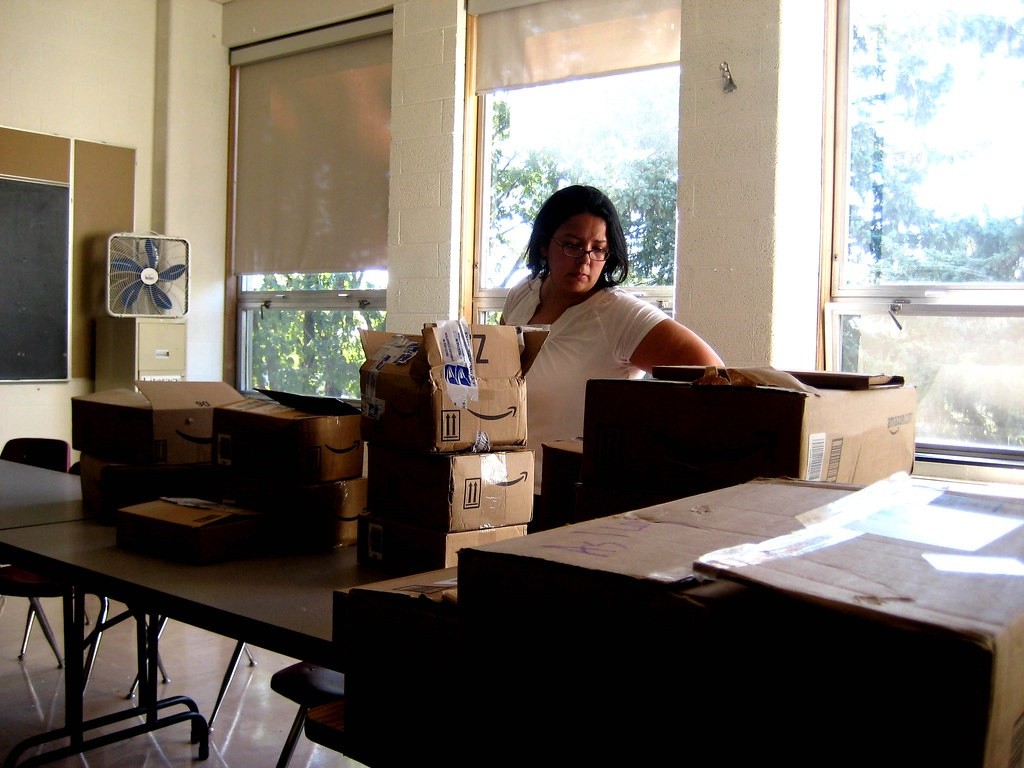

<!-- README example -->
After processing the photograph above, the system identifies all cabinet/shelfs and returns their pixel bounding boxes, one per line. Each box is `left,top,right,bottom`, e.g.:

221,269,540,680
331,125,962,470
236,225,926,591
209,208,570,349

96,317,187,394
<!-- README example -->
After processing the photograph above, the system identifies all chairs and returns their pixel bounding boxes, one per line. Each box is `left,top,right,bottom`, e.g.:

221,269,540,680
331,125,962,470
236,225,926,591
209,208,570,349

0,437,344,768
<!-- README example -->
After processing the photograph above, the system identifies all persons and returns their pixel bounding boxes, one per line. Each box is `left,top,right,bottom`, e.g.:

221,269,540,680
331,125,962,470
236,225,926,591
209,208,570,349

500,183,726,533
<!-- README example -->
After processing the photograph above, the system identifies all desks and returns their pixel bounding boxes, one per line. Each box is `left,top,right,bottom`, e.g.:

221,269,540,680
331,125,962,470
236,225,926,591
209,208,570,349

0,466,382,768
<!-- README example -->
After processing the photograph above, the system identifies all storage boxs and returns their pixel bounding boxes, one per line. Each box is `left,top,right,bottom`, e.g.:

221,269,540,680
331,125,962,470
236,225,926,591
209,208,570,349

70,317,1024,768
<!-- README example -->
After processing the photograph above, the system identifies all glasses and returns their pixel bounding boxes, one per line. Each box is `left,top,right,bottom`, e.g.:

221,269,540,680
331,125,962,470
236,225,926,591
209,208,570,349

552,236,611,261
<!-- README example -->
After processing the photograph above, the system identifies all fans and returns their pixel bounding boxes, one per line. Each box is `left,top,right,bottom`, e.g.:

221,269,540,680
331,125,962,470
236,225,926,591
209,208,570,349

104,230,189,319
